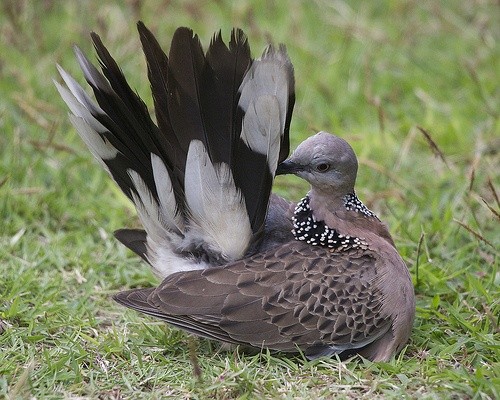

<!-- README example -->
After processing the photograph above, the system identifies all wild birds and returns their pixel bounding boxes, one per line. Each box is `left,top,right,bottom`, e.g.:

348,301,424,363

50,19,415,363
